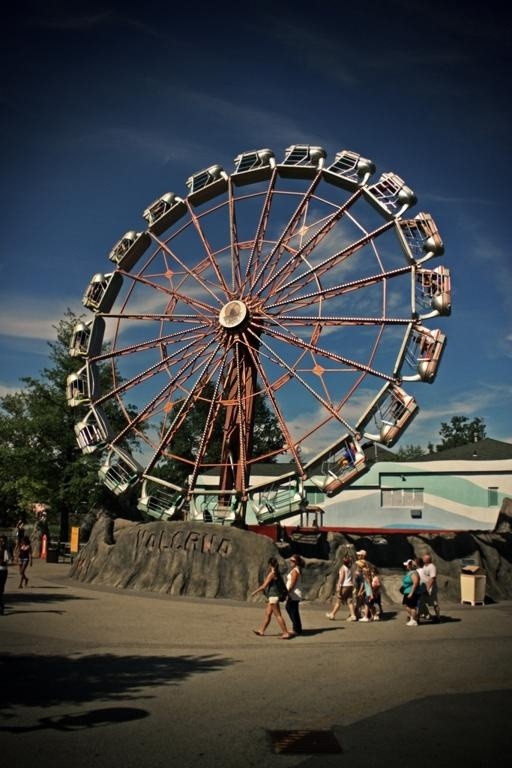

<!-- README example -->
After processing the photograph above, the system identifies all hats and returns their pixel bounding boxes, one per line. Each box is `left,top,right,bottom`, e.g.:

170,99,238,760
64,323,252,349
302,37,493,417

356,550,366,555
403,559,415,565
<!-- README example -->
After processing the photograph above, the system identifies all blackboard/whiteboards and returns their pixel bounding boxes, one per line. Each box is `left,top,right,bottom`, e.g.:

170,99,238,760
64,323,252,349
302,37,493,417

70,526,79,554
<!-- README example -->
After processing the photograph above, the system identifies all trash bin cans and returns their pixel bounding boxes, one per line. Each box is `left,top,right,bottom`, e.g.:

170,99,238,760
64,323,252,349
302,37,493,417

460,565,487,607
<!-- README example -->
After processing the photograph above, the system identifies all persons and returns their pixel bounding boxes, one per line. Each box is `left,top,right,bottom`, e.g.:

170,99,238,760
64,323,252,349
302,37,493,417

285,553,305,636
418,277,442,295
325,549,441,627
337,444,354,469
412,336,435,358
251,557,291,639
0,512,50,616
390,395,405,418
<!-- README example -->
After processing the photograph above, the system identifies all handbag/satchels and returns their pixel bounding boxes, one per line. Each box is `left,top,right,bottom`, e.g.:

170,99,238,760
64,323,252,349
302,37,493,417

400,587,404,593
280,580,288,601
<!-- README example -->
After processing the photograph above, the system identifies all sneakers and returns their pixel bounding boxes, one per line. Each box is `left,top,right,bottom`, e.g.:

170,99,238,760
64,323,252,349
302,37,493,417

406,613,441,626
346,613,380,621
18,579,28,588
326,613,334,620
